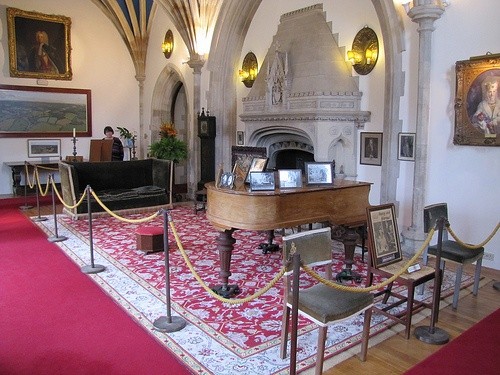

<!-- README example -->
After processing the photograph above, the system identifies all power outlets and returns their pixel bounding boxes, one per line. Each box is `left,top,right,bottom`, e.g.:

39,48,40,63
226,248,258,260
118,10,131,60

485,253,494,261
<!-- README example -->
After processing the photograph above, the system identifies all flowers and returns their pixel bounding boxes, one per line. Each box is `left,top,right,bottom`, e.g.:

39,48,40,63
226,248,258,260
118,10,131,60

157,119,178,142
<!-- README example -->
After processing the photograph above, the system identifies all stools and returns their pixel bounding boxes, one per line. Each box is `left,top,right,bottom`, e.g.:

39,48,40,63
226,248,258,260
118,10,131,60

134,226,166,255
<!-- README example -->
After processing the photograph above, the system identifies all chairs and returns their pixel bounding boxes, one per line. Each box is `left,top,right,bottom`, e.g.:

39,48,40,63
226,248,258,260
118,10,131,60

418,201,487,310
362,198,445,344
278,225,377,375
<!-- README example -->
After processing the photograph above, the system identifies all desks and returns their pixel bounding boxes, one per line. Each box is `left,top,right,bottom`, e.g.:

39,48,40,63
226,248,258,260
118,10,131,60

4,160,60,198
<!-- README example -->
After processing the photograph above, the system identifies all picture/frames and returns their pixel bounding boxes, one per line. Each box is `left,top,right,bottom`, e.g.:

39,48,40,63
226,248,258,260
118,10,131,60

397,132,416,162
365,203,404,269
0,84,92,137
6,7,73,81
243,157,303,191
27,139,61,158
453,53,500,146
305,161,335,186
237,130,244,145
359,131,383,167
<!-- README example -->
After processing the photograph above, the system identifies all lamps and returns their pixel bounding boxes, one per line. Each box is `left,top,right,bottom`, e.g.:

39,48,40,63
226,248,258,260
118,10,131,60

347,27,380,77
161,29,174,59
239,52,259,89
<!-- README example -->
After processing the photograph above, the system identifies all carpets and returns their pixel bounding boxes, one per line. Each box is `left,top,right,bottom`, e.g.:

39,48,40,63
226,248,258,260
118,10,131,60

27,199,492,375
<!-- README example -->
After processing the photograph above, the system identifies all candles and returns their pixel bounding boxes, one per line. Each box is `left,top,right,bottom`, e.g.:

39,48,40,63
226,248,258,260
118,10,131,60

72,127,76,137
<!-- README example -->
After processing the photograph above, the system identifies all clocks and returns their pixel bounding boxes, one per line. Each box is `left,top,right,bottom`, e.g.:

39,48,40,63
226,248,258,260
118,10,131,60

196,106,217,191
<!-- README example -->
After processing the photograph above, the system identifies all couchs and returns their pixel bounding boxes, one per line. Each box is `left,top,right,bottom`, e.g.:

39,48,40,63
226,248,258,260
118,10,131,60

58,156,173,222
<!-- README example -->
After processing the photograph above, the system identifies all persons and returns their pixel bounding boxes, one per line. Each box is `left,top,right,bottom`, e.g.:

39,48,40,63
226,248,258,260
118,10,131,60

255,174,269,188
288,171,298,183
102,126,125,161
376,225,390,253
